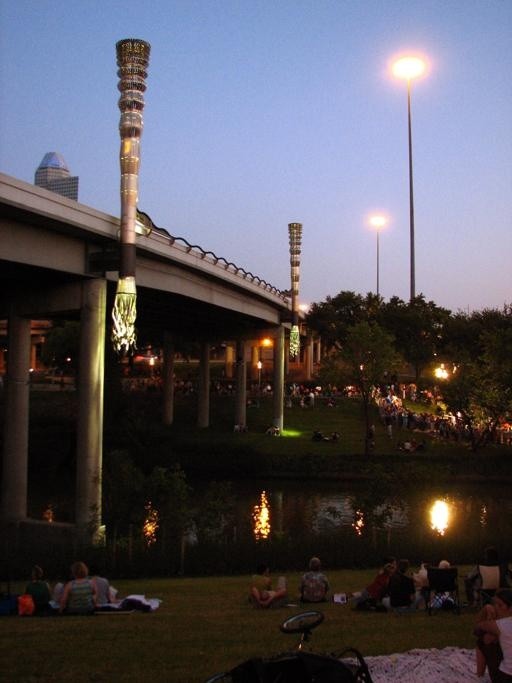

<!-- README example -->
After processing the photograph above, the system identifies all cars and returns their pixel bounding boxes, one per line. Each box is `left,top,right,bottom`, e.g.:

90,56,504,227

44,367,79,384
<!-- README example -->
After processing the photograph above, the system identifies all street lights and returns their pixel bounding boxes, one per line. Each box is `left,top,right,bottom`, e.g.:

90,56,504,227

258,361,262,385
370,56,425,299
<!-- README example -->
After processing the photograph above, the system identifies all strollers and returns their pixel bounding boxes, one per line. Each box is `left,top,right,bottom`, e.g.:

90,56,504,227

205,611,373,683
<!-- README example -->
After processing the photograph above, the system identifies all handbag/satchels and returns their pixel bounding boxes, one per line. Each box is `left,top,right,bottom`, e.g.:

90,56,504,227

0,590,37,617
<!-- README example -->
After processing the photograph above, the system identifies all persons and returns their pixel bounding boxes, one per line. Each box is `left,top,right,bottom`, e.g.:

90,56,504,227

246,572,289,608
233,422,249,432
312,429,343,442
118,372,347,408
351,555,453,614
347,378,511,454
271,424,280,437
23,560,122,617
297,556,330,603
470,588,512,682
463,545,498,608
